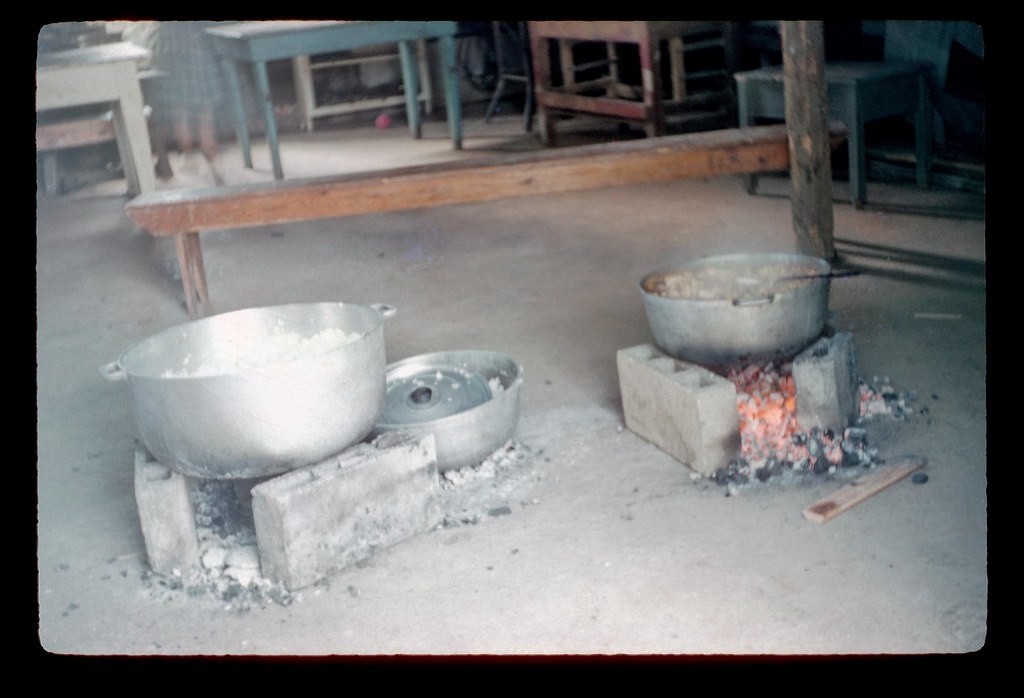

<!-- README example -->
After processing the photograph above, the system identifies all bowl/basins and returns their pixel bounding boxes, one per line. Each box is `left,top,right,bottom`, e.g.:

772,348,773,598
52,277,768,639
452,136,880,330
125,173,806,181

371,348,520,475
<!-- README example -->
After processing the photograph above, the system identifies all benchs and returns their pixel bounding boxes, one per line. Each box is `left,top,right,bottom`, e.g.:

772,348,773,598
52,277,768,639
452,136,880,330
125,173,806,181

122,121,849,321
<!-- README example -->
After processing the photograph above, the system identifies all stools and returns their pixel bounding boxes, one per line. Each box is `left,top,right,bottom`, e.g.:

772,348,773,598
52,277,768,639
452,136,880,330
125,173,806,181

732,61,930,212
204,21,463,179
526,20,733,139
36,41,158,198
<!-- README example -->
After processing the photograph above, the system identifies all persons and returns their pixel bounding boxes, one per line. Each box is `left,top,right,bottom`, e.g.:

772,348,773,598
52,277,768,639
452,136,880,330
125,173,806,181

150,20,225,185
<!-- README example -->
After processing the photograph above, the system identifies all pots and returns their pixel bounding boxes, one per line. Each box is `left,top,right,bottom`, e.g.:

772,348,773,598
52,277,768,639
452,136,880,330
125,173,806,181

638,253,831,369
101,301,398,480
